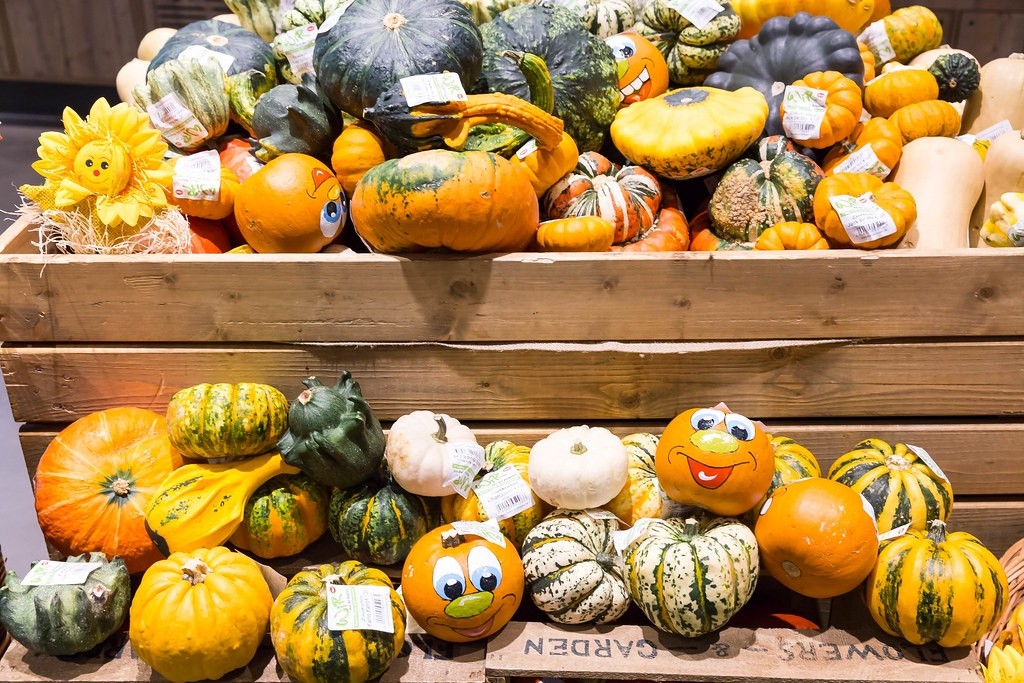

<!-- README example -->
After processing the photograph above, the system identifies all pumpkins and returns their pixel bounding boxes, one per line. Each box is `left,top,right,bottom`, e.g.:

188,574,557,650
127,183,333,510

0,370,1024,683
110,0,1024,253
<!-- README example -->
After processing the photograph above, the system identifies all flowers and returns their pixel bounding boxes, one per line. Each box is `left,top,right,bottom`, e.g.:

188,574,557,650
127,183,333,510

29,98,171,227
